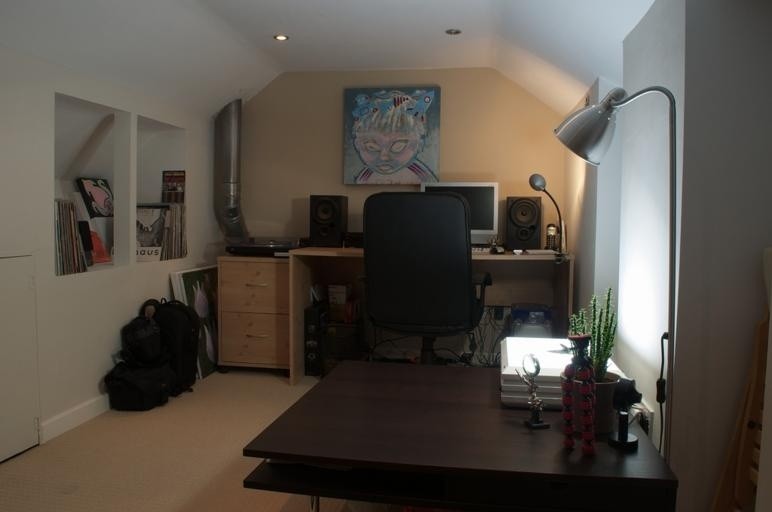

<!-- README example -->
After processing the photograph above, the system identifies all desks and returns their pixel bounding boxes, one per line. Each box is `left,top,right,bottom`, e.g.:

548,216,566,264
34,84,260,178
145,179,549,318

288,247,575,387
243,359,678,512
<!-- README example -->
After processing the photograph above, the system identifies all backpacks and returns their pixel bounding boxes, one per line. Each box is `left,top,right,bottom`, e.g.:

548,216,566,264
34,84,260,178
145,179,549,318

102,296,200,410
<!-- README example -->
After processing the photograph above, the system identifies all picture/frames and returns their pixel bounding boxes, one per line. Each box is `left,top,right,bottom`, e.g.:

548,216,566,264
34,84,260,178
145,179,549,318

170,265,218,380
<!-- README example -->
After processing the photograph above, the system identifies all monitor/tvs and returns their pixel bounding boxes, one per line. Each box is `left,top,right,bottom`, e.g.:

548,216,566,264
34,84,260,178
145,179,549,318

420,182,497,244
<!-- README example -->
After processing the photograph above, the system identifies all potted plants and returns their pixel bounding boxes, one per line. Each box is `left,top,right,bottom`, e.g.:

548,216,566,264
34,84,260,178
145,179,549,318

560,287,620,419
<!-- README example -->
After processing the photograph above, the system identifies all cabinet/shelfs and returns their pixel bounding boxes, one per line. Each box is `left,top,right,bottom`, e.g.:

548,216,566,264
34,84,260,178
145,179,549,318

216,255,290,379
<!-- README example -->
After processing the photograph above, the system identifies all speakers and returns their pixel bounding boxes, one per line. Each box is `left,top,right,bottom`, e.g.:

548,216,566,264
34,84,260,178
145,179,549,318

507,196,541,250
310,195,348,247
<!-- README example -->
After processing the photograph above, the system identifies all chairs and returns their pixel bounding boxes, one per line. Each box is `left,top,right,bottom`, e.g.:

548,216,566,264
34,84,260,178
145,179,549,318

361,191,492,365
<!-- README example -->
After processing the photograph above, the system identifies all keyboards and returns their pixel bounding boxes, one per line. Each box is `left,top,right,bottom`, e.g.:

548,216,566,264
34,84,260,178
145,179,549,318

471,247,490,255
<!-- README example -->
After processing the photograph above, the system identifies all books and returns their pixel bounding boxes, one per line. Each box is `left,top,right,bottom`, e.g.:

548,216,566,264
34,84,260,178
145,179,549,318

135,200,189,261
55,192,91,278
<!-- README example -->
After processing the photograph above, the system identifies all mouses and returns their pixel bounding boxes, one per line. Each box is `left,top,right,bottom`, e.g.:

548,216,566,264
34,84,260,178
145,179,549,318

492,247,505,255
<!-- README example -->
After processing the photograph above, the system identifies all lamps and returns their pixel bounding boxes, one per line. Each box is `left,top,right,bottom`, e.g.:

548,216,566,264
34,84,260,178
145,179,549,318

552,86,676,467
529,174,570,265
